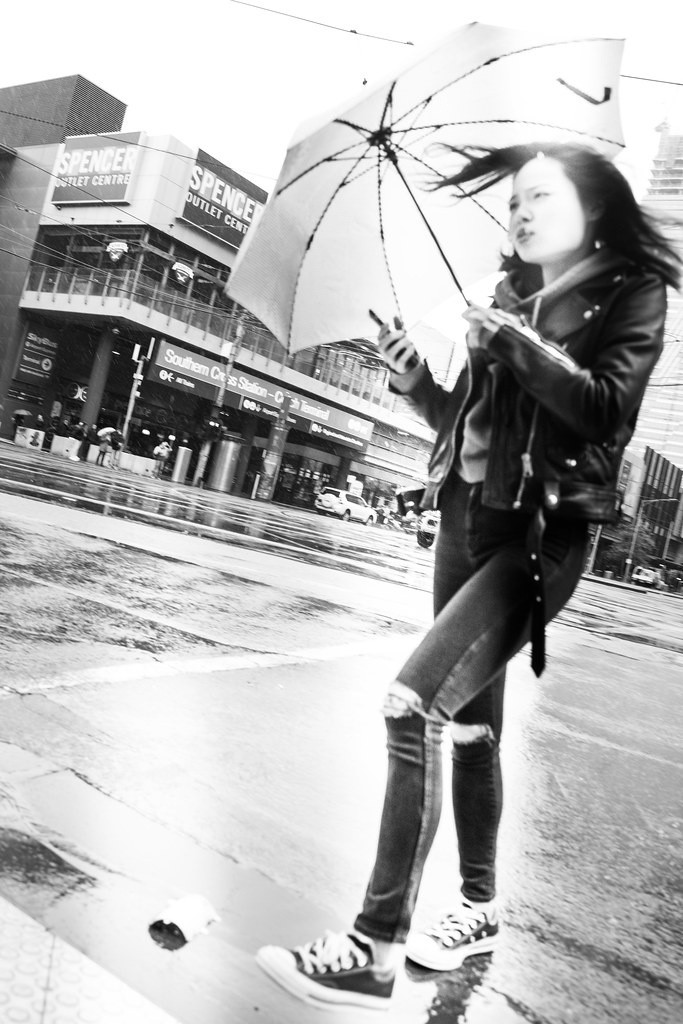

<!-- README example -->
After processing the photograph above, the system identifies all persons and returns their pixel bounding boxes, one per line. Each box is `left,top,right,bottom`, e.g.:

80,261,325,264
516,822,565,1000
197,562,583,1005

248,142,683,1006
11,413,173,480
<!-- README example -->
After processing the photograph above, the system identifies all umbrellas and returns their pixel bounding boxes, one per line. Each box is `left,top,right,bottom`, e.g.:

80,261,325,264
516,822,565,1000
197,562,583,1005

14,409,33,420
225,18,629,355
97,427,116,437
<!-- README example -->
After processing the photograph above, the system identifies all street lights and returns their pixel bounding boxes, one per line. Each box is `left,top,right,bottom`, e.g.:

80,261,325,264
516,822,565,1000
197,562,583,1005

623,498,679,582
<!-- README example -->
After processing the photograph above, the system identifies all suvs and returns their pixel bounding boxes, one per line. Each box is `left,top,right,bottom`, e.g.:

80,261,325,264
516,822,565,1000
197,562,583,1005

314,487,378,527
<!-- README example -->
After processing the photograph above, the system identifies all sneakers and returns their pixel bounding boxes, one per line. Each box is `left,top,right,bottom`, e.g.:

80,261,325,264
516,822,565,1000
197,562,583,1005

256,933,393,1010
405,899,501,971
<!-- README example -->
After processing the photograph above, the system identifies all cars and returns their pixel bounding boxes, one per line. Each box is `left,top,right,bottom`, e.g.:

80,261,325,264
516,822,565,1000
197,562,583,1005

631,565,661,586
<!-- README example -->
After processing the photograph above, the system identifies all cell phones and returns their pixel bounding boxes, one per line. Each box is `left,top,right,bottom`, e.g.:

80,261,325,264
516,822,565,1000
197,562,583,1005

368,309,421,367
